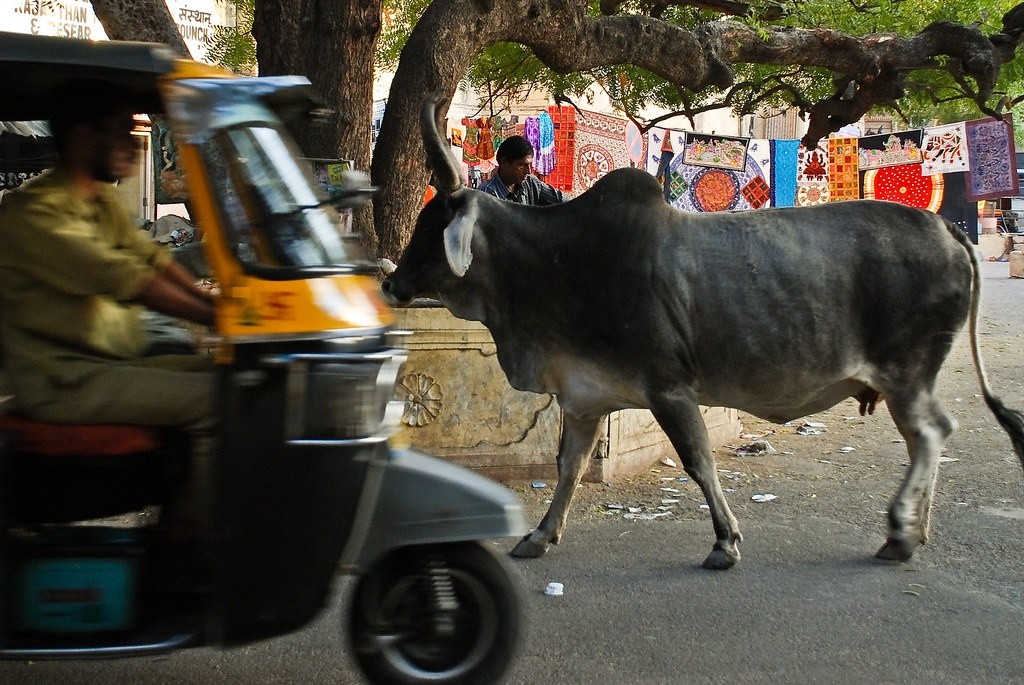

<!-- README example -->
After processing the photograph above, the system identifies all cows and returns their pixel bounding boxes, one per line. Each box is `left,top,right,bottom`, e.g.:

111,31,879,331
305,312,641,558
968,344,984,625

379,88,1024,570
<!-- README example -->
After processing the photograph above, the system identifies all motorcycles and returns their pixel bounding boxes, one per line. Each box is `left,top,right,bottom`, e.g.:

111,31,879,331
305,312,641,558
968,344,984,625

0,28,527,684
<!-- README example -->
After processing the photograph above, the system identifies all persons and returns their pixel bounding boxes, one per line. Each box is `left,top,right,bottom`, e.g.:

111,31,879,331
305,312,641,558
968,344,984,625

478,135,569,206
0,85,281,625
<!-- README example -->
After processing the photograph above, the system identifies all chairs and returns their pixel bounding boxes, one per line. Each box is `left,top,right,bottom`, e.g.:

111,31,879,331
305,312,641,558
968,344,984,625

0,408,165,516
